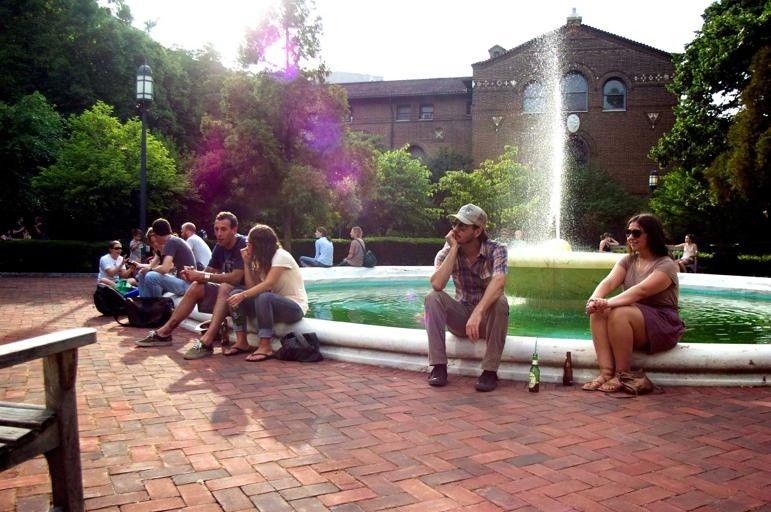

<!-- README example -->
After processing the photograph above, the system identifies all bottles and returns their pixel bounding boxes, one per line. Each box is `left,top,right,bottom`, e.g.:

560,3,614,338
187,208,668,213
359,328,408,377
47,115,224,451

528,352,540,394
227,305,243,327
563,352,574,386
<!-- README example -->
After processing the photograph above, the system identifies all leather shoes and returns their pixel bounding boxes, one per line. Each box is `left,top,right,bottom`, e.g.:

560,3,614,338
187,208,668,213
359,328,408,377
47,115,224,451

428,364,447,387
474,371,498,391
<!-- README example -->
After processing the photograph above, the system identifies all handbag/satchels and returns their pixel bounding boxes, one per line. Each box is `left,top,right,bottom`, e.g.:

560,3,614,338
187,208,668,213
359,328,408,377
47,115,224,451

114,296,174,329
275,331,322,361
363,250,377,268
617,368,665,398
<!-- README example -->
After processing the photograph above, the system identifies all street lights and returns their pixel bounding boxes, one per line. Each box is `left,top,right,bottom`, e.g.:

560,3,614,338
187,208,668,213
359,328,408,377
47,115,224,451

132,57,154,228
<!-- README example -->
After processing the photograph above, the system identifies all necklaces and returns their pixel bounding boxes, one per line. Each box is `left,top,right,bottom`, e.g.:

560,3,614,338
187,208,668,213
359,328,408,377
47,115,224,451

639,256,649,273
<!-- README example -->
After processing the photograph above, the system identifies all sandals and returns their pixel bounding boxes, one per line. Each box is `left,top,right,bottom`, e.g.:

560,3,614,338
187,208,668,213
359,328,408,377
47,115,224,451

597,370,623,393
581,369,615,392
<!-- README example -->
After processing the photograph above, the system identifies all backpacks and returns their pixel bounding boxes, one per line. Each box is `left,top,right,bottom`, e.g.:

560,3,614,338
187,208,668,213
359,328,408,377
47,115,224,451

93,283,127,317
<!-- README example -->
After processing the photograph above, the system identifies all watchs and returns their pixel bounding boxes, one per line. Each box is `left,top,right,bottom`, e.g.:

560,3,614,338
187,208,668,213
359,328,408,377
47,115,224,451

242,291,249,298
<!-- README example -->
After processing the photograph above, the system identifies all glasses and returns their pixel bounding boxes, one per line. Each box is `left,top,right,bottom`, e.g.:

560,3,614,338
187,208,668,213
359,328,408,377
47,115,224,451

450,220,470,230
112,247,122,250
624,229,643,239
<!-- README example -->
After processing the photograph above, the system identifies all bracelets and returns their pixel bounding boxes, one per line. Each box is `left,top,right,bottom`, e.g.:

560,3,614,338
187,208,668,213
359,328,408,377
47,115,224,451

203,272,212,282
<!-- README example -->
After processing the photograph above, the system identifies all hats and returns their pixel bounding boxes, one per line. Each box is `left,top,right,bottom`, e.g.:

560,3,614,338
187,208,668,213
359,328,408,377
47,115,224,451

448,203,487,231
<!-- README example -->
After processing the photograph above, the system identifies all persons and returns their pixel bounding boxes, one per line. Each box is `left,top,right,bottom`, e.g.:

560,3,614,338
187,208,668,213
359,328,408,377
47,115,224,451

597,232,620,252
300,227,334,267
423,202,509,392
511,230,528,248
336,226,367,266
222,224,311,362
94,216,213,314
665,233,699,274
582,213,684,392
495,229,512,245
136,210,249,362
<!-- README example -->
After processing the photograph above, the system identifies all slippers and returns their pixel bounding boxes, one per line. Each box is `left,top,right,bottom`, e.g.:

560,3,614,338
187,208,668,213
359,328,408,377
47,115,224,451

245,350,276,362
222,346,251,356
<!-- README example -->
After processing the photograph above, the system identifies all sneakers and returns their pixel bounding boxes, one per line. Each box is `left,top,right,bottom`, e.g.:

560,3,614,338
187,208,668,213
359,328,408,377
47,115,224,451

134,330,173,347
183,339,213,360
